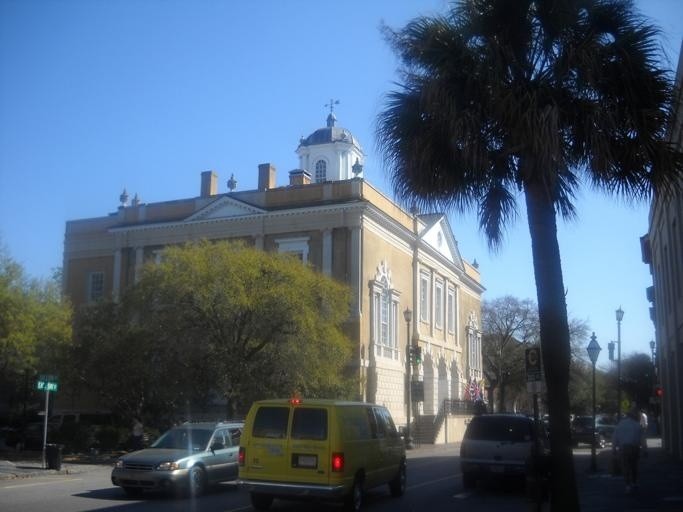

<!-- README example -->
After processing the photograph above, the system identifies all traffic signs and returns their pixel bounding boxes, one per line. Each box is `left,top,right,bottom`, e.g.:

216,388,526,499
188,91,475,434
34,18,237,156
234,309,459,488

35,380,57,392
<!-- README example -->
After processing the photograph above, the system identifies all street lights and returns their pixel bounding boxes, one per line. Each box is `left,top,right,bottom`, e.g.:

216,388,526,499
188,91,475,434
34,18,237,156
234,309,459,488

402,304,413,441
585,331,602,466
648,339,654,363
613,303,624,425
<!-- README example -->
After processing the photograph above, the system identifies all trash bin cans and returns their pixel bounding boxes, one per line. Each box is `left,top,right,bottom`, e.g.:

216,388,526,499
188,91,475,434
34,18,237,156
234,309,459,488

45,444,64,471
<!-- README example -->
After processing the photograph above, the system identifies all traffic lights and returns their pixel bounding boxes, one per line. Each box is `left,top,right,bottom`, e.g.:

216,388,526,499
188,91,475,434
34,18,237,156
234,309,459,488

413,345,422,366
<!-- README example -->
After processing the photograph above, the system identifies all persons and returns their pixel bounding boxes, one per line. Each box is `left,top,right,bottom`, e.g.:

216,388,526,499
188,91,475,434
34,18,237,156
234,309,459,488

610,409,650,493
638,407,647,434
128,414,144,453
86,433,102,464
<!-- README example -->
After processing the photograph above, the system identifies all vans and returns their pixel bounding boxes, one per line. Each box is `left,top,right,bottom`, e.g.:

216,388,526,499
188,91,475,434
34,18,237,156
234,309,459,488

236,396,409,509
457,409,547,488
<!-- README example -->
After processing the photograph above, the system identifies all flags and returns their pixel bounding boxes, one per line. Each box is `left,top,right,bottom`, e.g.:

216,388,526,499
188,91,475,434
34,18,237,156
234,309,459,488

465,378,471,401
471,378,478,400
478,380,483,402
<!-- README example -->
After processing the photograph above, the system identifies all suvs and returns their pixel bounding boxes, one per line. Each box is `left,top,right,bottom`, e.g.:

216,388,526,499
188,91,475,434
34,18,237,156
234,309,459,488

108,418,247,496
571,413,621,449
